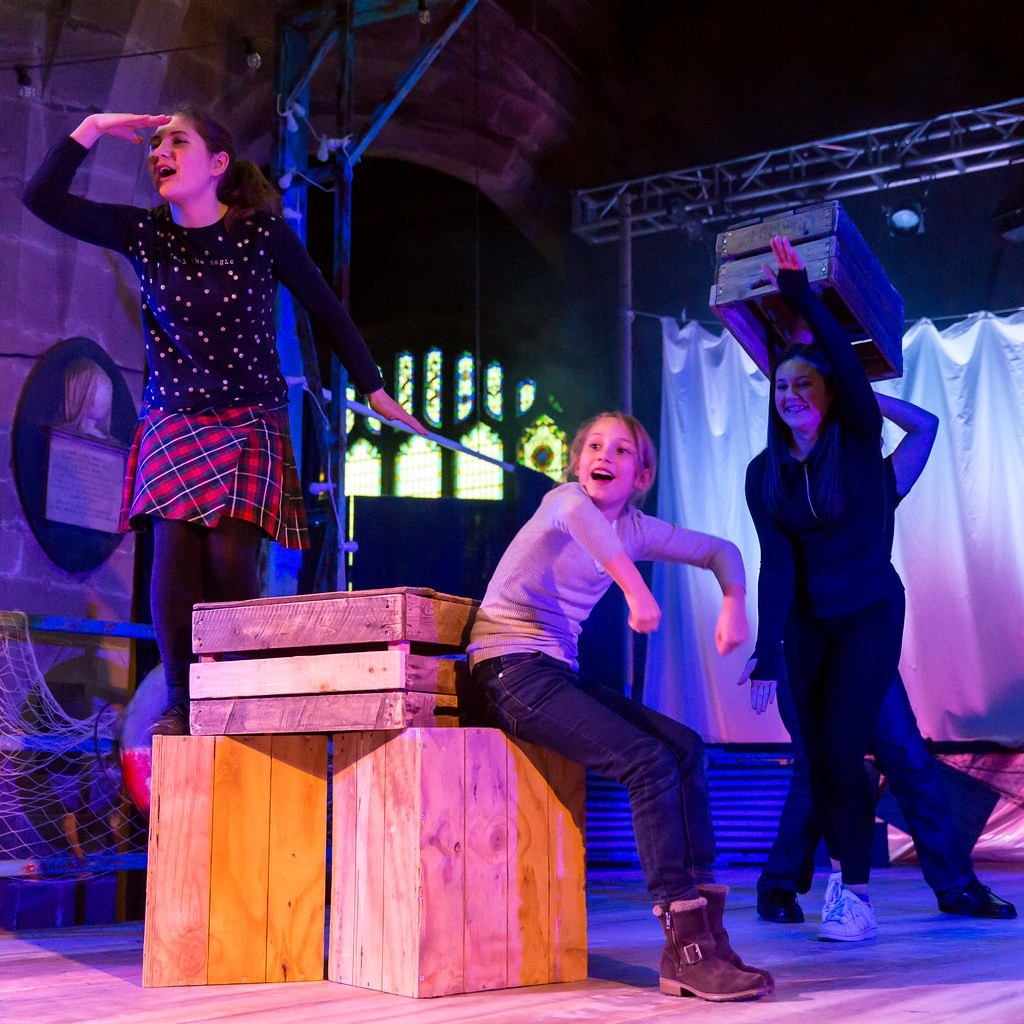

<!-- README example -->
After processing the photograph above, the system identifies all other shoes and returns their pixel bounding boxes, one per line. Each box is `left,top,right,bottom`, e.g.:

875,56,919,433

756,889,805,923
939,884,1018,919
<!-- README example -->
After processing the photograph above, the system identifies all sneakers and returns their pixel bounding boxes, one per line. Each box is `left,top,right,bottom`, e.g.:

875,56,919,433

818,888,878,942
821,871,843,924
145,703,189,740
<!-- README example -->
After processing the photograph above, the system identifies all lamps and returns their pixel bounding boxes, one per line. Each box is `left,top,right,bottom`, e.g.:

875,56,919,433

882,181,929,238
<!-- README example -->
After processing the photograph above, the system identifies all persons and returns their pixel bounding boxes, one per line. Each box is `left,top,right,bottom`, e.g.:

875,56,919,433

738,237,1018,940
467,414,774,1001
22,106,428,733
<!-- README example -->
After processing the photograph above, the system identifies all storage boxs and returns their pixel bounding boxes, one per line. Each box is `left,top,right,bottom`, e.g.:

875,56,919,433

143,586,589,1000
710,200,905,382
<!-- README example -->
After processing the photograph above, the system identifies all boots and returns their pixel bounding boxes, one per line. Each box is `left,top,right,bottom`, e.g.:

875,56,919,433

695,883,775,991
653,897,767,1001
